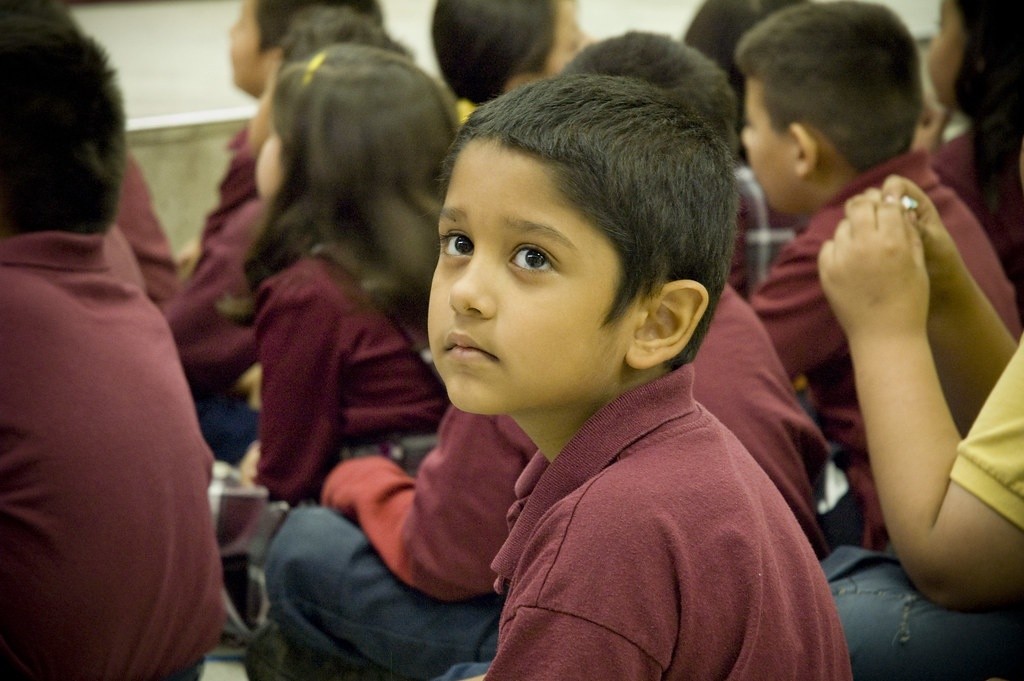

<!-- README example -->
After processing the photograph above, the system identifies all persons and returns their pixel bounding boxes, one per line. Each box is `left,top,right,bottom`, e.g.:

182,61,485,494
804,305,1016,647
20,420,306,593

427,76,854,681
812,175,1024,681
1,0,1024,578
0,11,223,681
